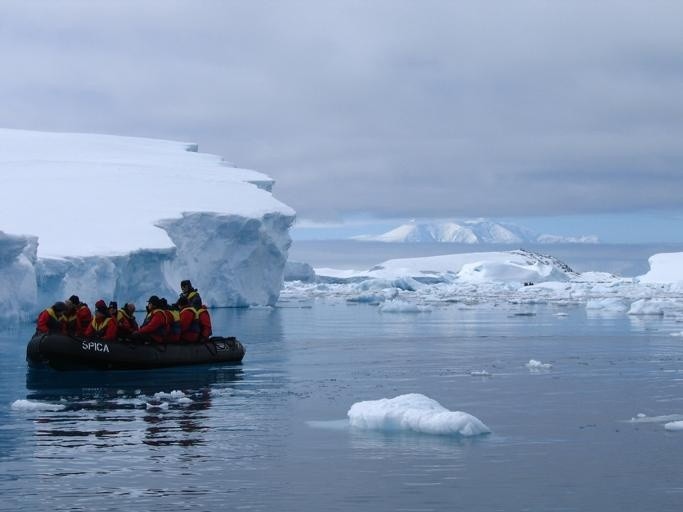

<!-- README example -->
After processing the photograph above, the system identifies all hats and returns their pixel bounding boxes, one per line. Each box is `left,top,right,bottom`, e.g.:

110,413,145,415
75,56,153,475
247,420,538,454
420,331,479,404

178,296,188,305
146,295,160,304
95,300,106,310
181,281,190,286
126,303,136,313
54,301,66,310
70,295,79,304
109,302,117,309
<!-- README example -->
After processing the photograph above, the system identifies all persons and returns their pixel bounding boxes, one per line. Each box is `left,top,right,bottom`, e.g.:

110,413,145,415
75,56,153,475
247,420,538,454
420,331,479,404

175,280,201,308
37,295,212,344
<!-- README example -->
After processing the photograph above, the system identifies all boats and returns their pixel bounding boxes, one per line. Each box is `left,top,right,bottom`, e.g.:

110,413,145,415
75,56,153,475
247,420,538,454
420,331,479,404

24,333,248,371
